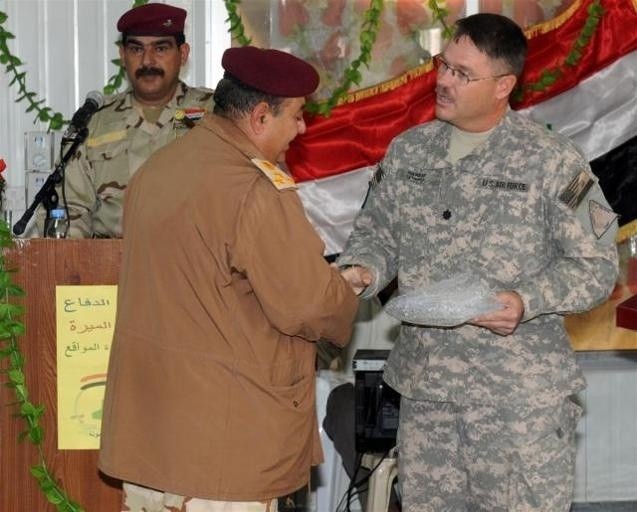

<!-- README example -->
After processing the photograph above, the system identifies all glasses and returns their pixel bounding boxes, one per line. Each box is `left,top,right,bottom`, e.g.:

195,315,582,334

432,52,513,87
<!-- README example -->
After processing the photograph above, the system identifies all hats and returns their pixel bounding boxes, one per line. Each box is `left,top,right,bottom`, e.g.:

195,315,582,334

222,46,319,97
117,3,187,37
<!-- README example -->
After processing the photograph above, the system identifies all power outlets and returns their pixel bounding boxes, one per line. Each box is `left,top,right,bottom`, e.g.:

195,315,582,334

22,131,66,208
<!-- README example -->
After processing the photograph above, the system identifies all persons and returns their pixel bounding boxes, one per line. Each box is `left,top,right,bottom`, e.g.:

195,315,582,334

54,2,215,240
97,47,361,512
327,12,621,512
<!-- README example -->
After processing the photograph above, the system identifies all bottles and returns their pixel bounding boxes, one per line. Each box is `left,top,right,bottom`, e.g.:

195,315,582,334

46,209,69,238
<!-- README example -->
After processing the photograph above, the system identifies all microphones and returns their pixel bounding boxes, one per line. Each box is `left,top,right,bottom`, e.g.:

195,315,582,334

73,89,105,129
175,109,195,129
